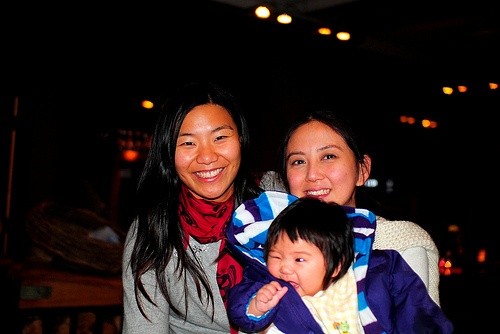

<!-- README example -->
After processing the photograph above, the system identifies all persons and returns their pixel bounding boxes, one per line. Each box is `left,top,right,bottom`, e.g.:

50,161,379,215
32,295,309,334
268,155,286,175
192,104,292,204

237,111,440,334
121,88,286,334
225,191,454,334
